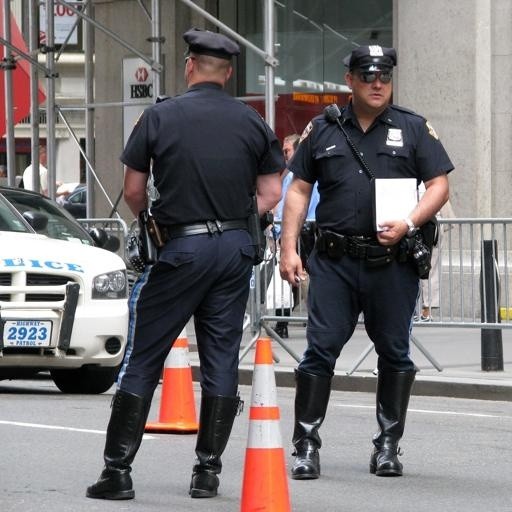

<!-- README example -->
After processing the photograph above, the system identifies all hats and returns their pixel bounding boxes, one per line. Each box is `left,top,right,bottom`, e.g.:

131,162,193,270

342,44,397,73
182,24,240,60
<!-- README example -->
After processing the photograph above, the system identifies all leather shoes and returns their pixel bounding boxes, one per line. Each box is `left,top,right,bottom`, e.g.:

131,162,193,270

271,326,288,337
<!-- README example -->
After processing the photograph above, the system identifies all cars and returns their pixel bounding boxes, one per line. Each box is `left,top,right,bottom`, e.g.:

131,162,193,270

0,185,120,254
58,186,86,218
0,187,130,396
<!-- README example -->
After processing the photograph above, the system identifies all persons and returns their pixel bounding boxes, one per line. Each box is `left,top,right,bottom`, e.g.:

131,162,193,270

277,44,456,480
22,144,70,202
412,181,455,323
266,133,321,340
86,25,286,501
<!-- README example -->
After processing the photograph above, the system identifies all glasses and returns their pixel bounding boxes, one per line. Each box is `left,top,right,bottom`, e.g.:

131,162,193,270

360,72,392,83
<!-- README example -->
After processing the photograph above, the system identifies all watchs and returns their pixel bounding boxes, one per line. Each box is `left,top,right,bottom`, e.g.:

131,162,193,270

406,218,416,237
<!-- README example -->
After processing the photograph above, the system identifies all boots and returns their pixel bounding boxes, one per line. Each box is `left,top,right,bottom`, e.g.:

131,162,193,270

187,393,240,499
289,369,333,481
368,371,416,478
86,388,152,499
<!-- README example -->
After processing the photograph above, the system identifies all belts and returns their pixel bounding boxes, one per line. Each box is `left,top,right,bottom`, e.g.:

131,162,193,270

168,218,248,239
315,230,409,257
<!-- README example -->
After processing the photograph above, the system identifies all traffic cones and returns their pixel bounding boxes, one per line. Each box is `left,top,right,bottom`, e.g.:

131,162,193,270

239,338,292,512
145,325,201,433
